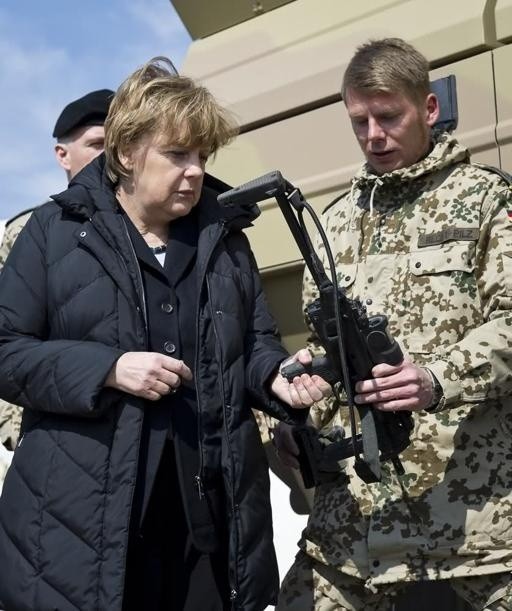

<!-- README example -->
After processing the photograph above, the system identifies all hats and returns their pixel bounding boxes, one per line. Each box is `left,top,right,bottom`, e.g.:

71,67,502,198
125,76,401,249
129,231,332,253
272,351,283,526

53,90,115,137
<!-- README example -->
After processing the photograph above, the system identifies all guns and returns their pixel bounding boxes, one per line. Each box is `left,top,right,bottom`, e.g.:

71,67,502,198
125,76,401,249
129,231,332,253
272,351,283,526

216,170,414,476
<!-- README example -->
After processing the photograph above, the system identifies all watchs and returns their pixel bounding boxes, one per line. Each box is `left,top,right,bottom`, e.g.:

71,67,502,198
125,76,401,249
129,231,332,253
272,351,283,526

426,366,443,408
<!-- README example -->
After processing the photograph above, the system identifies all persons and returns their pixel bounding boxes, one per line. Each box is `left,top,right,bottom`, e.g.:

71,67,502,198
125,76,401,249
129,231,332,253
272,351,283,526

0,89,114,491
1,59,336,610
275,36,510,609
